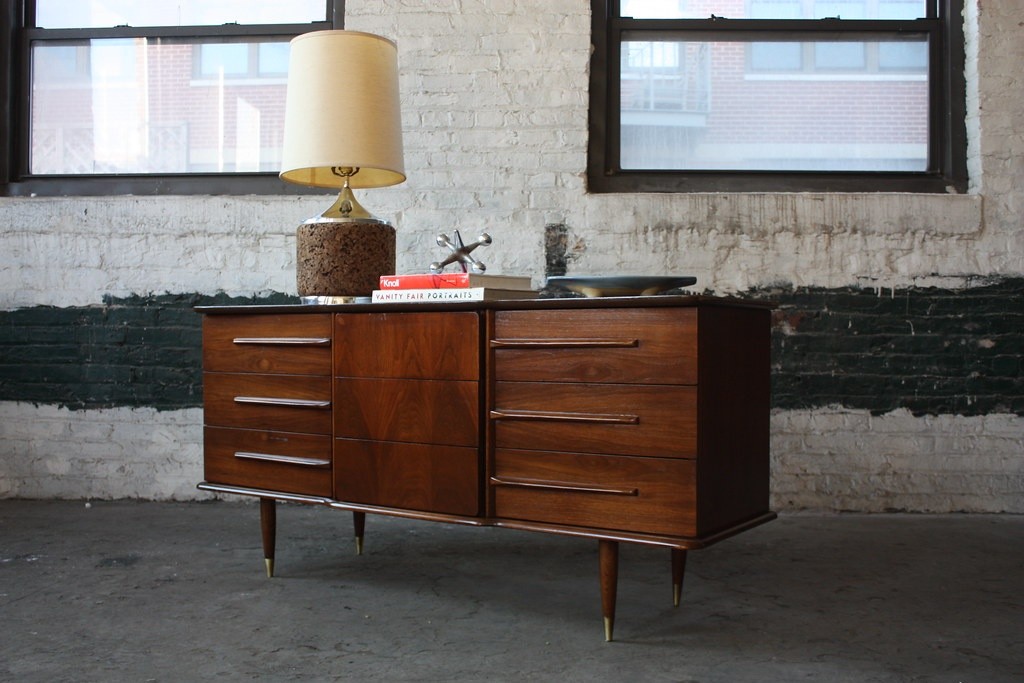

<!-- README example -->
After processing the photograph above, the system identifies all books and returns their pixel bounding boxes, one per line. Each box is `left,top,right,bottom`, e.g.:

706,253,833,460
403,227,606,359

370,270,542,304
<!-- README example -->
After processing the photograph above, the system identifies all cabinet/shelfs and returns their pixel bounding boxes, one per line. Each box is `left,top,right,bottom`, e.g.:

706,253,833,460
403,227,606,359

194,295,777,643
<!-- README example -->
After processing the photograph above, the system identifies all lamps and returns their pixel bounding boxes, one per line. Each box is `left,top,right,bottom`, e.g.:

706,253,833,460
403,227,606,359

278,30,407,305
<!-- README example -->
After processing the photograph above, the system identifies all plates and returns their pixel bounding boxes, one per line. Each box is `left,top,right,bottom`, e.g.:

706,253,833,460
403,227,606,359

547,276,697,298
